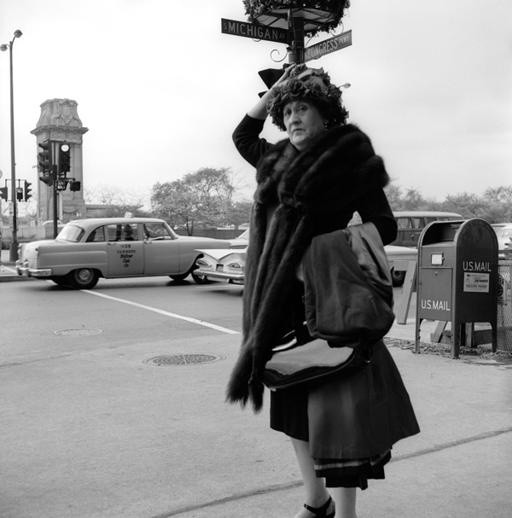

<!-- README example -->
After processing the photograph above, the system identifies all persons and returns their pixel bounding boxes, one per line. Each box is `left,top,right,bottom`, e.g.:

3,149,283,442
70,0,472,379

222,65,423,518
124,224,134,240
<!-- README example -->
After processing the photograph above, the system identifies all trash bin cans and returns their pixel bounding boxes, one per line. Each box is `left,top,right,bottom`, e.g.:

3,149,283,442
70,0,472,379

416,220,498,359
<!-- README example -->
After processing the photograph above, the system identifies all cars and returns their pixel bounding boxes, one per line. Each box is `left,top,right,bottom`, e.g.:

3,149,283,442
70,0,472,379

15,217,250,290
347,210,511,288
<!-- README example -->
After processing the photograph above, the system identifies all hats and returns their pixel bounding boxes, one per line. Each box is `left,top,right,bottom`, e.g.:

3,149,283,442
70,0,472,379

269,64,348,131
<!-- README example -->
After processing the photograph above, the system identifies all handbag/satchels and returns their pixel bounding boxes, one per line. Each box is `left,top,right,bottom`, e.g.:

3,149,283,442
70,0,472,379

259,277,360,392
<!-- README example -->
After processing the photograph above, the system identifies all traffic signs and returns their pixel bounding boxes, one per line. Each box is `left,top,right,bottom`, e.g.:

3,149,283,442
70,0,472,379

221,18,352,62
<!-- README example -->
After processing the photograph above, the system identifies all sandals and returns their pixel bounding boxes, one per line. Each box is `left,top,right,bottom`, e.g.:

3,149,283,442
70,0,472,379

303,495,336,518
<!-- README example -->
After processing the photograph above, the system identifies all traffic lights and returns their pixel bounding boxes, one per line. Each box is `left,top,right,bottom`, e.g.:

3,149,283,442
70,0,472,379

37,138,80,191
0,180,32,202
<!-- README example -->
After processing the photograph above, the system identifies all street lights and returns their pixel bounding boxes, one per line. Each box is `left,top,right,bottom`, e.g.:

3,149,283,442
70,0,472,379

0,29,23,262
338,83,351,89
